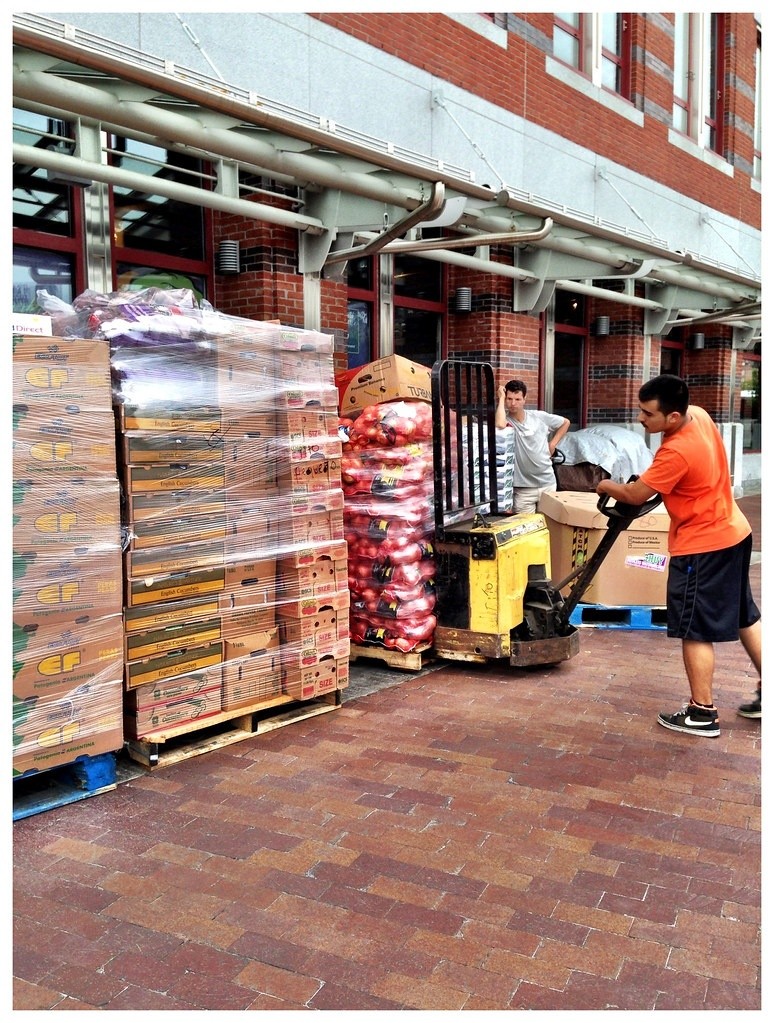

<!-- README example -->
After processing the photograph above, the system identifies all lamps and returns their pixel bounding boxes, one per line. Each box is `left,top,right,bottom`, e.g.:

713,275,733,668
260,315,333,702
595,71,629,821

596,317,609,335
695,334,705,349
219,240,240,272
455,287,471,312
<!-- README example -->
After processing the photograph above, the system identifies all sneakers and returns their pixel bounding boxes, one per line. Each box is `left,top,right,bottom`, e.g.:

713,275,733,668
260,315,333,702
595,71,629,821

738,681,762,717
657,699,720,737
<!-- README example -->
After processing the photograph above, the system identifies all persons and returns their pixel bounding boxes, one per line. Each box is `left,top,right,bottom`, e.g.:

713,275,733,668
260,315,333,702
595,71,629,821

596,375,762,737
496,380,570,513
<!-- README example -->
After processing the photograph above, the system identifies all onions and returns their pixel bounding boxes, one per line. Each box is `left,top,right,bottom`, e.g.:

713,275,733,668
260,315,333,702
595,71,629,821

336,399,460,654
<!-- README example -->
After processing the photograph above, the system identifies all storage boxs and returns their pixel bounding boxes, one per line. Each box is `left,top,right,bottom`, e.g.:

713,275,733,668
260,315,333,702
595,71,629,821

12,304,349,776
335,354,441,420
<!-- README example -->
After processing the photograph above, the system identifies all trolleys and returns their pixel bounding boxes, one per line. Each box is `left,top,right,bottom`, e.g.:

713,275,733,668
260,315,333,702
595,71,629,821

338,360,664,674
